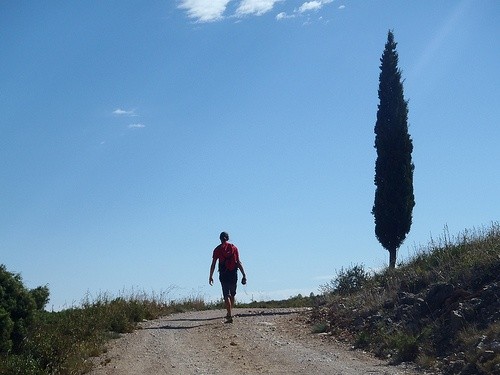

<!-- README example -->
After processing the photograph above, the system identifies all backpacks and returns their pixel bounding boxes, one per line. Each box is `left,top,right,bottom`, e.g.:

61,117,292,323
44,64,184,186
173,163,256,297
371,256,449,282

218,244,236,272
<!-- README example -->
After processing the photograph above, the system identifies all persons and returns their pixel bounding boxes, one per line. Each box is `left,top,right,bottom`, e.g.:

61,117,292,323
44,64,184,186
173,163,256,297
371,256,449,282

209,232,246,323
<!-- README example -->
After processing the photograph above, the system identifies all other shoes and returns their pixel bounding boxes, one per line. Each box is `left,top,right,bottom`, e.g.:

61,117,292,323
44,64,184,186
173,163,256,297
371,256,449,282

225,313,232,322
